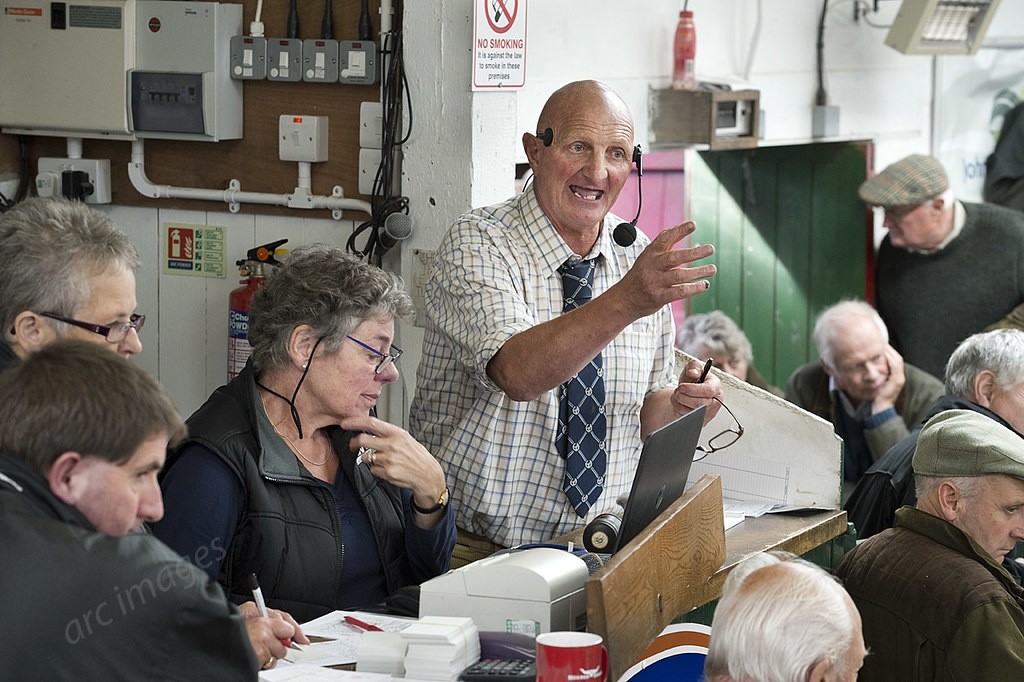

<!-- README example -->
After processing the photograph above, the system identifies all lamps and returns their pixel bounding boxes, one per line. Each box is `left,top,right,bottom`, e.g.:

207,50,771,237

885,0,1001,55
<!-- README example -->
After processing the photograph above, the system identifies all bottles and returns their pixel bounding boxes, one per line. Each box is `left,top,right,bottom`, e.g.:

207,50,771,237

673,11,696,86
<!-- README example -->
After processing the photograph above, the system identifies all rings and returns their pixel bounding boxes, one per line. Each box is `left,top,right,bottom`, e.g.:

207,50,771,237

262,656,274,669
370,450,375,466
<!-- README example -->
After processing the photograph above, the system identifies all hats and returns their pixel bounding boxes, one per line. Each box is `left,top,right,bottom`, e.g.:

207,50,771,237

911,409,1024,476
858,154,949,208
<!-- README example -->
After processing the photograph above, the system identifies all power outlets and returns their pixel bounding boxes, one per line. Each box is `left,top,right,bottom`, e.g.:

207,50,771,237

37,156,111,204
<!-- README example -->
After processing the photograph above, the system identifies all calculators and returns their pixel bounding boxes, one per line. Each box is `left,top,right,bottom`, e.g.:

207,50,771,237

461,658,536,682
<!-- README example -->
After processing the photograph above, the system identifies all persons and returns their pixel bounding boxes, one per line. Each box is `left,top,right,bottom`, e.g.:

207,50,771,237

702,552,865,682
409,79,725,575
856,154,1024,384
0,338,259,682
834,408,1024,682
676,310,784,400
144,243,457,626
782,298,1024,587
0,198,311,672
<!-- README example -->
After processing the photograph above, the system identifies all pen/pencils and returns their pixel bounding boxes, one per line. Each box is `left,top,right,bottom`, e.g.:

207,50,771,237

248,573,269,619
344,616,382,631
697,358,713,384
280,639,303,652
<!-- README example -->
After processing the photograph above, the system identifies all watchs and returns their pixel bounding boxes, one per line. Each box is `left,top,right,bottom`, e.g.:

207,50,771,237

413,488,449,515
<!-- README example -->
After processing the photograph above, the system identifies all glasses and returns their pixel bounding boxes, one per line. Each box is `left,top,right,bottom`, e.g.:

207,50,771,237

345,336,403,373
11,313,146,343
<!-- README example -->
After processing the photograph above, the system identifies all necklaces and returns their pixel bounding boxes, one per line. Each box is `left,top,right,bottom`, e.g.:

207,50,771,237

258,392,331,466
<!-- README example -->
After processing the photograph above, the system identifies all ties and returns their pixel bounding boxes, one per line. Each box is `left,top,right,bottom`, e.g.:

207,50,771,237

557,259,613,518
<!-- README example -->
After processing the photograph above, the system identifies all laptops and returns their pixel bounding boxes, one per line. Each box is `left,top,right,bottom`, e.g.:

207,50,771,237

487,405,708,572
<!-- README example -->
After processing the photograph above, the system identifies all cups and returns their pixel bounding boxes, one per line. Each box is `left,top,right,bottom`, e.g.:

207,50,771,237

535,630,610,682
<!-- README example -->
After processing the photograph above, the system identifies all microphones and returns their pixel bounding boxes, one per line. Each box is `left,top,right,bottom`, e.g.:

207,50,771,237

374,212,414,256
612,176,641,246
